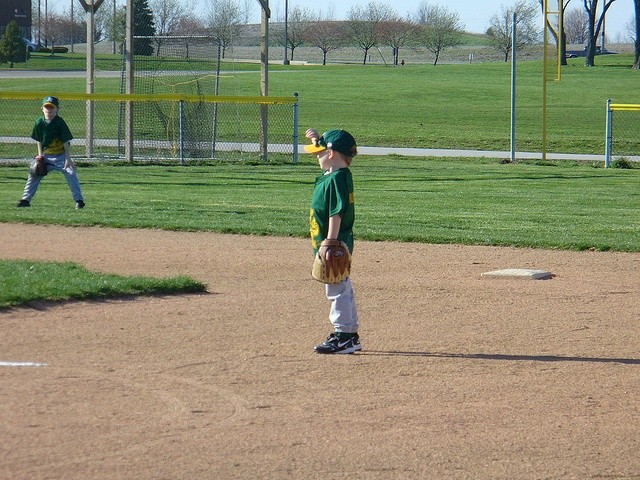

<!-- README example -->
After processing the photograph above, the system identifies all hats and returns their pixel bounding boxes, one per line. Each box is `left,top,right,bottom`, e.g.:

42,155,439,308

41,96,59,108
303,129,357,157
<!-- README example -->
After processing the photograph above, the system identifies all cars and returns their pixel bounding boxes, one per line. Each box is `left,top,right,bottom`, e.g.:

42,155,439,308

22,38,36,52
565,45,619,59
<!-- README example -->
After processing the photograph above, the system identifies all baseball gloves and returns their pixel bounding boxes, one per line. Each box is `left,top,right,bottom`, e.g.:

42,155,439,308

29,156,47,176
312,239,351,284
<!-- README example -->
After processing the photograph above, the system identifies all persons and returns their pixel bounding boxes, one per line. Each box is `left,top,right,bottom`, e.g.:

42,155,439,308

303,129,361,354
15,96,85,209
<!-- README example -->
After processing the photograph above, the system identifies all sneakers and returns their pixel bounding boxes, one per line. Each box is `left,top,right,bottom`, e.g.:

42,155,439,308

353,332,362,351
75,200,85,209
313,332,355,354
16,200,30,207
